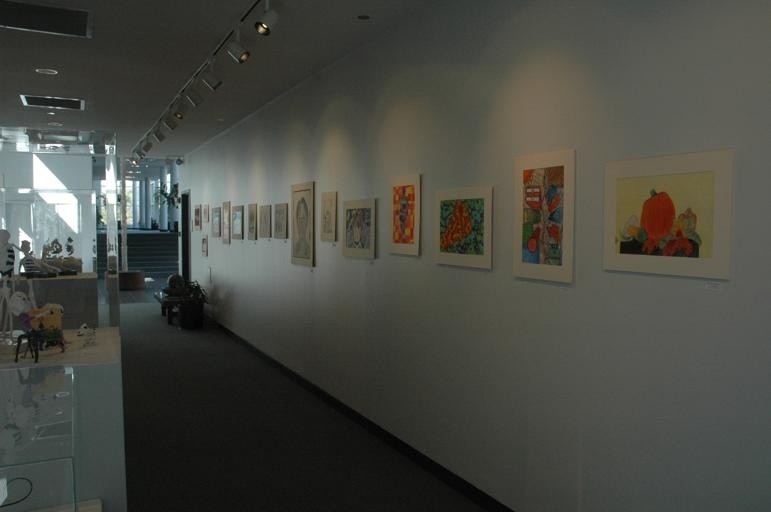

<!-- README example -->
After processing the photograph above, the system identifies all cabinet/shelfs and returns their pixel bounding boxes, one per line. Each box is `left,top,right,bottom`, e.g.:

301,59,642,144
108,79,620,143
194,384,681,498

3,327,128,511
0,271,98,328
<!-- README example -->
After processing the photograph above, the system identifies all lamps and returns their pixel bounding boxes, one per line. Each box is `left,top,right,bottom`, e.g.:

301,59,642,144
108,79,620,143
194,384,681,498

128,9,276,166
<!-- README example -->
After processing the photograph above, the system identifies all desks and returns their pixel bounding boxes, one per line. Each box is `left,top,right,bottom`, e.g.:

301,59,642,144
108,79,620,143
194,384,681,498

154,291,204,328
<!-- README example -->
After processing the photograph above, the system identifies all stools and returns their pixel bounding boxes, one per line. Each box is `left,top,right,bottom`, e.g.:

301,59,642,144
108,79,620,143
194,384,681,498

104,270,146,289
